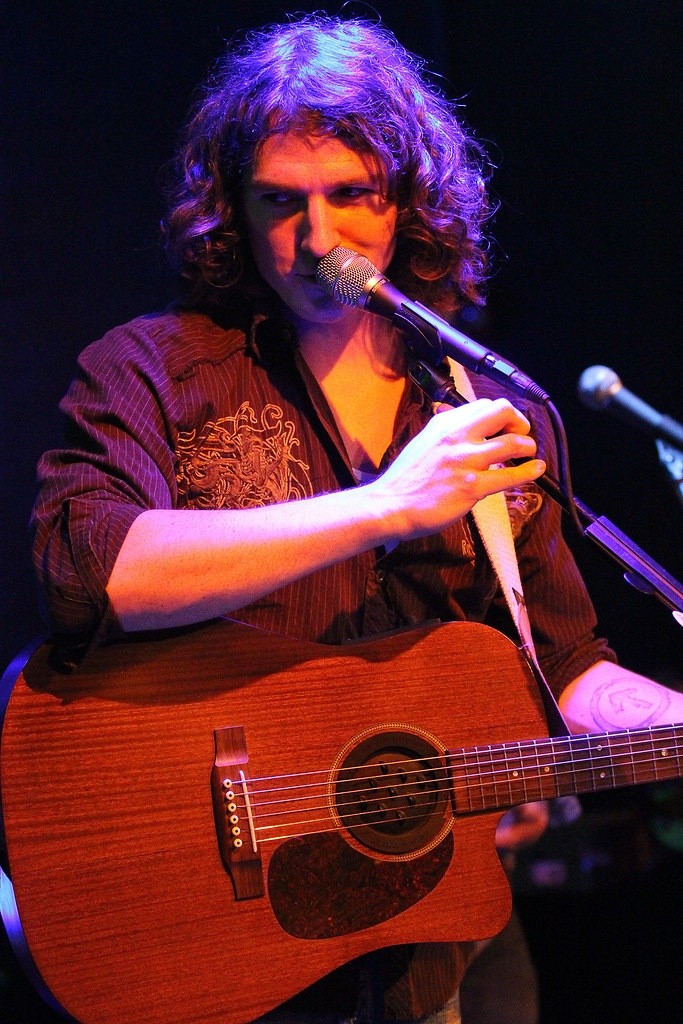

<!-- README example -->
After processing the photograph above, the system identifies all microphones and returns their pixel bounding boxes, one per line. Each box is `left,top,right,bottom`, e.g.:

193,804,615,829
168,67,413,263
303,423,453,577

314,247,551,405
578,363,683,454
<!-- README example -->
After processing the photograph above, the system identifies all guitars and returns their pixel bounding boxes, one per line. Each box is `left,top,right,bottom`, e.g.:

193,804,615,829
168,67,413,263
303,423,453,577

5,609,683,1023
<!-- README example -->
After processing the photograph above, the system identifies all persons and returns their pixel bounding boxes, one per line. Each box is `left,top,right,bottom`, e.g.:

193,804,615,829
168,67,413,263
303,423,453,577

14,12,683,1024
